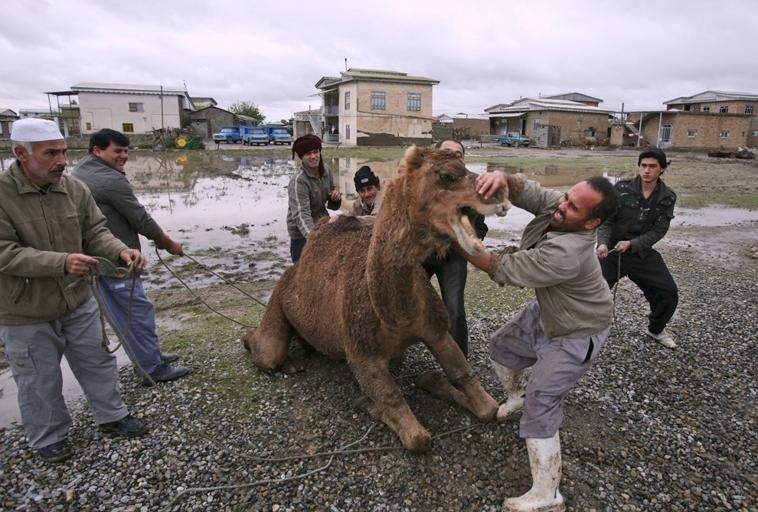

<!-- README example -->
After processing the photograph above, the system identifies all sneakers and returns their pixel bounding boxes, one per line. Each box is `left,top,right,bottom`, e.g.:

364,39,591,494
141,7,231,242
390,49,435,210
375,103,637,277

646,325,678,348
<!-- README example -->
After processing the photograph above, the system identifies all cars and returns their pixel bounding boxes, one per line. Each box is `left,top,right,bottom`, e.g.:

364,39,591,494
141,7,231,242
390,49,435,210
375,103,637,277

494,131,533,148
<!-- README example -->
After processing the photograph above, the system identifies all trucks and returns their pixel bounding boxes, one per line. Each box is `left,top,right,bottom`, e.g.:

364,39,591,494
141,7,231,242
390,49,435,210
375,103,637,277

213,125,293,146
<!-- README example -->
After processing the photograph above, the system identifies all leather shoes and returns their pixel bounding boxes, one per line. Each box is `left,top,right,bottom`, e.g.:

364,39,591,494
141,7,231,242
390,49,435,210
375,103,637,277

37,438,72,464
133,352,179,374
143,362,189,386
99,412,147,437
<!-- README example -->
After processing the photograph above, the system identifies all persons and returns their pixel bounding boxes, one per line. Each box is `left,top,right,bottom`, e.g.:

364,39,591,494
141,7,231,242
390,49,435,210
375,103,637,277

597,145,679,349
287,133,343,264
69,128,191,386
454,168,622,512
347,165,385,230
0,118,149,462
420,137,488,363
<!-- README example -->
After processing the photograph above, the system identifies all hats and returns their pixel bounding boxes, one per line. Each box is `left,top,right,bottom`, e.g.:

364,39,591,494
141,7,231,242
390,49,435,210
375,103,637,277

354,166,379,191
292,133,322,158
10,117,64,142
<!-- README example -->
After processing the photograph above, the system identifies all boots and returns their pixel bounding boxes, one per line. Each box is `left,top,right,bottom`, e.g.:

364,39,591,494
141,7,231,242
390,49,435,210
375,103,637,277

488,356,530,421
503,429,567,512
